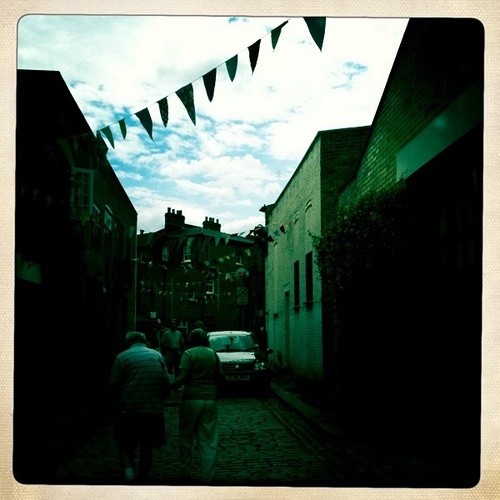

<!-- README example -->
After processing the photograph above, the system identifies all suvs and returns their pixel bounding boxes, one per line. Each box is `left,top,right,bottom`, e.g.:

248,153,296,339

206,330,273,394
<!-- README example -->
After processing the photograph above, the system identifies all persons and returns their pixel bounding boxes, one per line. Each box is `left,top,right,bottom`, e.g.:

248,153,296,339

109,331,171,485
174,329,222,486
161,323,184,376
